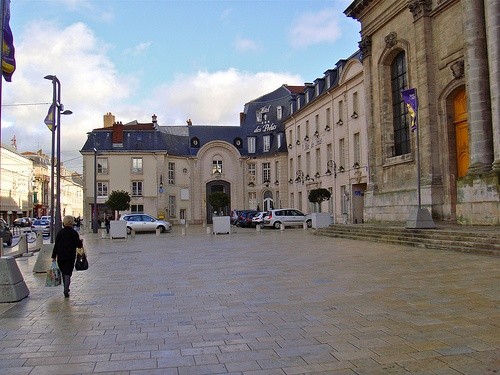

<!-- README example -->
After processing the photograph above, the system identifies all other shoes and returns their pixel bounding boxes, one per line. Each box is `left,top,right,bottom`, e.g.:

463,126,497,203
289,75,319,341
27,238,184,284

68,289,70,292
64,291,69,298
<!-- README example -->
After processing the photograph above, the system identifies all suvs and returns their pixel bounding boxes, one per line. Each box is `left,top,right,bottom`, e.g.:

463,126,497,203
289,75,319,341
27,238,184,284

230,206,312,230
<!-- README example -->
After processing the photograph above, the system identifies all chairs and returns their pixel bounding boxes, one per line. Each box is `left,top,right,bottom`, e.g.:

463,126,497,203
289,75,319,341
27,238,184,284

288,211,292,215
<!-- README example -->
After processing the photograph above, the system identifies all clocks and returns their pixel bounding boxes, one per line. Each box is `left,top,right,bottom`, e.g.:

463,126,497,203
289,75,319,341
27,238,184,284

193,140,198,146
236,140,241,145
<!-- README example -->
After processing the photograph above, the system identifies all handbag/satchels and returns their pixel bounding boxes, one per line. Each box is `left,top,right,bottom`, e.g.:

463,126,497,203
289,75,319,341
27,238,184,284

45,259,62,286
75,248,88,271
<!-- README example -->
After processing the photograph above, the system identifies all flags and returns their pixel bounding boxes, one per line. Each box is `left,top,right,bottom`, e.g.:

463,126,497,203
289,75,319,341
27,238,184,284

45,103,57,132
0,0,16,82
402,88,418,133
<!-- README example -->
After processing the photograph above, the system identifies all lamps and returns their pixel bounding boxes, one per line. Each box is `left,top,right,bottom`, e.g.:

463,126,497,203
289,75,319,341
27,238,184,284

152,114,157,123
214,165,221,177
265,180,271,187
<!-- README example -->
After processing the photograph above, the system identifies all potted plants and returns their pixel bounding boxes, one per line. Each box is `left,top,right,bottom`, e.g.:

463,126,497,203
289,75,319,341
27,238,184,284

105,190,132,239
295,171,320,182
324,125,330,131
288,178,293,184
314,131,319,136
296,140,300,145
274,180,279,185
308,188,332,229
304,135,309,141
325,169,331,176
208,190,231,235
337,119,343,125
351,112,358,119
338,166,344,172
353,162,360,169
289,144,292,147
248,182,256,187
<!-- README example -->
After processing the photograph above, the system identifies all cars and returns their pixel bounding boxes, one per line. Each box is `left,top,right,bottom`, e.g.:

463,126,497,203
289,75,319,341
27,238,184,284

0,217,13,247
12,215,52,237
119,213,173,234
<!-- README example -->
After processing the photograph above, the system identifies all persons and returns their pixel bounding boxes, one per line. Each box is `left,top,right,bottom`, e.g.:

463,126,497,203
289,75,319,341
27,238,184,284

51,215,83,298
105,215,111,233
76,216,83,230
74,216,76,222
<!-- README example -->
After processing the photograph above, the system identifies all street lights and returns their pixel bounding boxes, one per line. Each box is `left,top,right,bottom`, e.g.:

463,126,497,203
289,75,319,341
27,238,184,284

43,74,74,235
